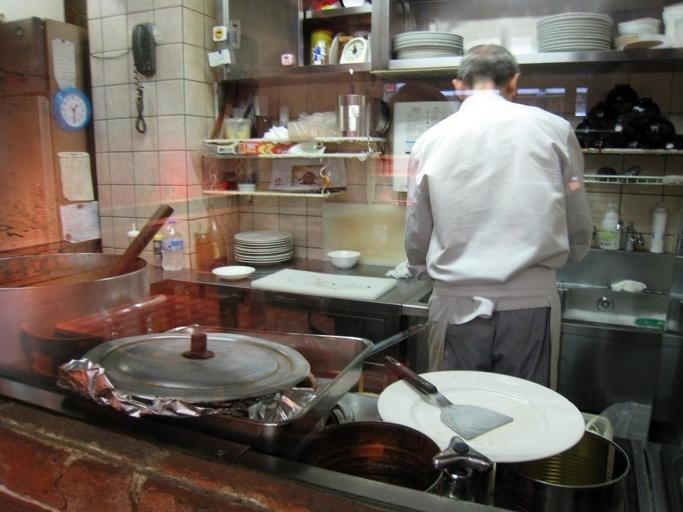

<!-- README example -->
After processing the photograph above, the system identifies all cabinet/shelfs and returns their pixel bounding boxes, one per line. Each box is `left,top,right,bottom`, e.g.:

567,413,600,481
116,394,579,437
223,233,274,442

203,1,683,201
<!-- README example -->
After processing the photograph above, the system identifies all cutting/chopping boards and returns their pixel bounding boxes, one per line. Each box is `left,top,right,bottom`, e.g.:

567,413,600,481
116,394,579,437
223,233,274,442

251,268,398,301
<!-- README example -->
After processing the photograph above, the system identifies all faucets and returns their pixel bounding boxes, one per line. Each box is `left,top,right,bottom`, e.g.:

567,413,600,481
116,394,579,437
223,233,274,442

616,219,644,250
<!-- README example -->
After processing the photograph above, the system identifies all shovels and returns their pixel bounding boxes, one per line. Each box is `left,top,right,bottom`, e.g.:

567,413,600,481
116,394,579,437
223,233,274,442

384,355,513,440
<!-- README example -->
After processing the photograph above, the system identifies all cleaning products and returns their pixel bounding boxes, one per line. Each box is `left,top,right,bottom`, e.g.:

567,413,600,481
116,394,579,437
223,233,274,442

651,203,669,252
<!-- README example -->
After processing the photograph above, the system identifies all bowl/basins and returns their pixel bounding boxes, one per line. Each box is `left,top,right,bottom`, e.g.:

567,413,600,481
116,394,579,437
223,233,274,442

327,250,361,269
617,18,662,33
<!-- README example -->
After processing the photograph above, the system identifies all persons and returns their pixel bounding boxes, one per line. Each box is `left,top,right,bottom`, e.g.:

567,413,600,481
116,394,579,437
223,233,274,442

404,43,595,391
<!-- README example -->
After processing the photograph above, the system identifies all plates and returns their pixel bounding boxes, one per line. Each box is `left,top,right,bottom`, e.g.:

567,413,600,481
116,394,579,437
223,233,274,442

377,370,586,463
535,12,614,51
233,230,295,266
612,34,670,50
211,265,256,281
238,184,257,188
393,31,464,58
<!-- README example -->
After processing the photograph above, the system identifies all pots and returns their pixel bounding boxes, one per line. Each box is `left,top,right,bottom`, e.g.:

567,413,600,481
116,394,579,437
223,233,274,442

0,252,149,377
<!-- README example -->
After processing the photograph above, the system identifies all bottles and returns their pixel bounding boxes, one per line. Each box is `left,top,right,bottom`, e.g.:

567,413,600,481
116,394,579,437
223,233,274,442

127,223,143,259
153,218,185,271
338,93,370,137
576,83,676,149
193,201,227,272
649,201,669,253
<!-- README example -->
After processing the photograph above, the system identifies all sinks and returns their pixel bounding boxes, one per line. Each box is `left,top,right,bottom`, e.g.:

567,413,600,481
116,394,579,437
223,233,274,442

556,247,683,299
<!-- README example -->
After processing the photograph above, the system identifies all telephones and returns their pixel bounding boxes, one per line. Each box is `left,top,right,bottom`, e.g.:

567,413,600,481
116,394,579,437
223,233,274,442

132,25,156,74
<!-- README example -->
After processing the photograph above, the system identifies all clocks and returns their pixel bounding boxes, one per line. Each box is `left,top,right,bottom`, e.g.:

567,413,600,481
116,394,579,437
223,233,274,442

53,87,92,132
